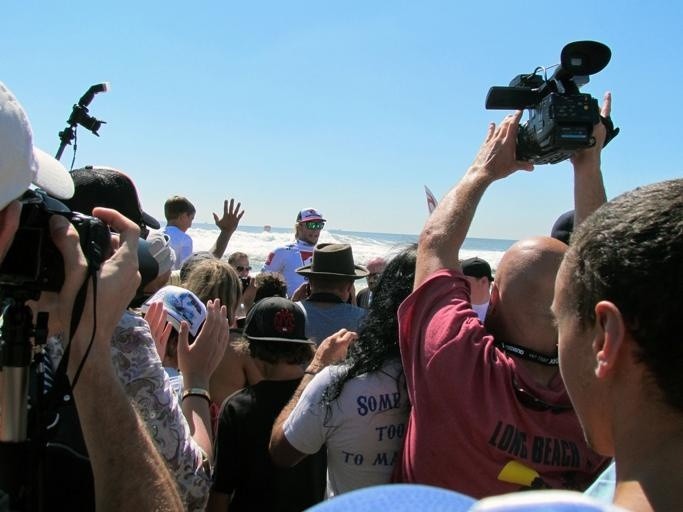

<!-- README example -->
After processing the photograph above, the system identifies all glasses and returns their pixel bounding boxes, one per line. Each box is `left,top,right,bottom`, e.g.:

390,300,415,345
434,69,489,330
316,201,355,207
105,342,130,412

300,221,324,229
232,261,252,272
366,272,382,280
512,371,573,415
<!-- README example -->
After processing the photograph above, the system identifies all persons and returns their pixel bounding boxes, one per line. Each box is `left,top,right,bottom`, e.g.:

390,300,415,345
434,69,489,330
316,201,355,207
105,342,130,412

1,86,683,510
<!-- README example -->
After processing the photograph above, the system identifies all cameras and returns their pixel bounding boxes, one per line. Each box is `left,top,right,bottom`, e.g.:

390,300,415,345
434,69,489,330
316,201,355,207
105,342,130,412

239,276,250,294
68,82,108,136
0,192,159,367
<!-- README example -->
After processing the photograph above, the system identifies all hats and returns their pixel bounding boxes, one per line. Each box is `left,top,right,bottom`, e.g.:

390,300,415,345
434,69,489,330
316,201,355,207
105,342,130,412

243,294,315,344
550,210,573,245
295,209,325,224
460,257,495,281
0,81,75,212
295,243,371,280
66,165,217,338
